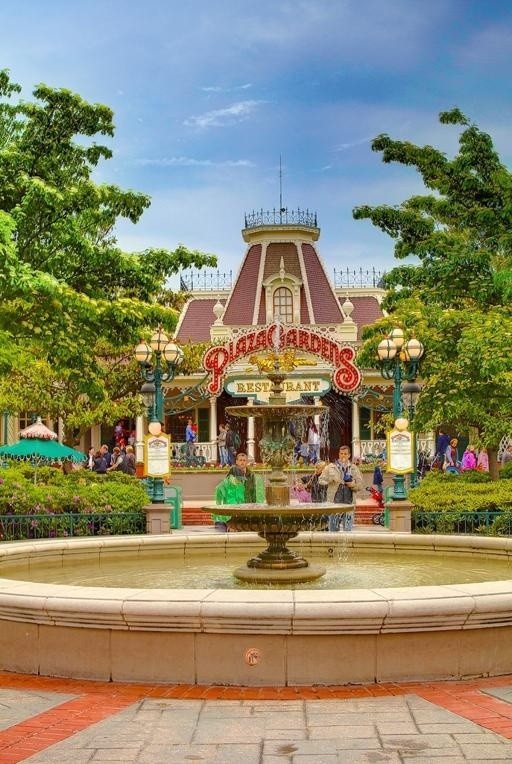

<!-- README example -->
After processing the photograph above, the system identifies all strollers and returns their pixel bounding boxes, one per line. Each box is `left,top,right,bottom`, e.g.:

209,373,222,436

417,447,433,478
364,486,368,497
174,444,208,468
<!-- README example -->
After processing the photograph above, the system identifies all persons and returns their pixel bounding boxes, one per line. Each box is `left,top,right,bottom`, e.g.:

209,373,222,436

295,446,363,531
216,423,237,468
226,453,256,502
185,419,198,443
84,421,136,475
502,444,512,468
436,429,489,475
373,459,384,493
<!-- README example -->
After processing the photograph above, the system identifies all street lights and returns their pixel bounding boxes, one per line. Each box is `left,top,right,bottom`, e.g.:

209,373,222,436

373,325,422,503
401,375,421,490
132,324,183,505
140,373,159,502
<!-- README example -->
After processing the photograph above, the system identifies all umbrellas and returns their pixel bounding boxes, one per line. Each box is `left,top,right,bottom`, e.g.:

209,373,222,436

0,416,88,484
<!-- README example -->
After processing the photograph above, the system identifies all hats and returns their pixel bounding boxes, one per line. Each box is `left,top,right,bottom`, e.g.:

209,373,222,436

229,465,248,481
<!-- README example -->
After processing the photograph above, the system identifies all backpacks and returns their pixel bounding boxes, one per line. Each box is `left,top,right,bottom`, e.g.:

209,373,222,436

227,431,241,450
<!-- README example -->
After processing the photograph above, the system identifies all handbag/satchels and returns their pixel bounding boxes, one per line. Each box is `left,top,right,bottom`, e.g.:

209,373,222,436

334,484,353,505
